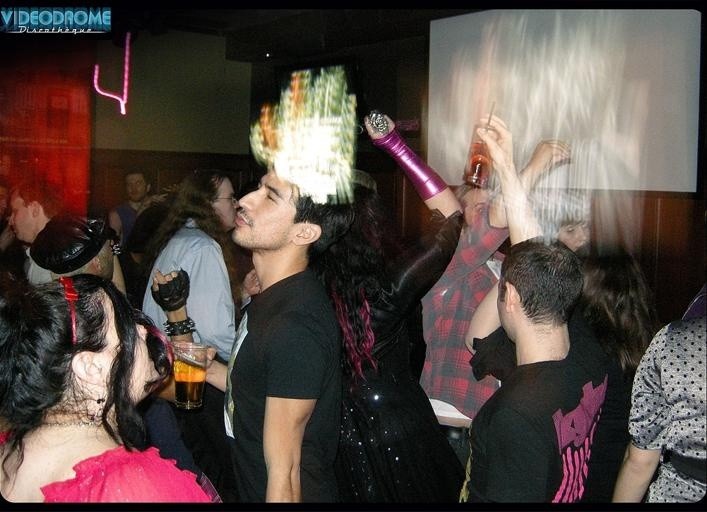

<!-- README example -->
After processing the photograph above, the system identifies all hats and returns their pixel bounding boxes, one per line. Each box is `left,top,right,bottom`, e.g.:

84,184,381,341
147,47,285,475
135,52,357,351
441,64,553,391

29,205,116,274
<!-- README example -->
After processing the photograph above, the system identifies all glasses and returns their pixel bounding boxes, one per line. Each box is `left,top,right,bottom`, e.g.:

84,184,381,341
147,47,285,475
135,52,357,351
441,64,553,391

216,196,237,206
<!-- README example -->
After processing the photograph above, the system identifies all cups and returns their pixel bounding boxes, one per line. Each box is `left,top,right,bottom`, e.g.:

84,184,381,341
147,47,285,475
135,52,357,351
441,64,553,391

169,339,209,410
462,122,500,191
